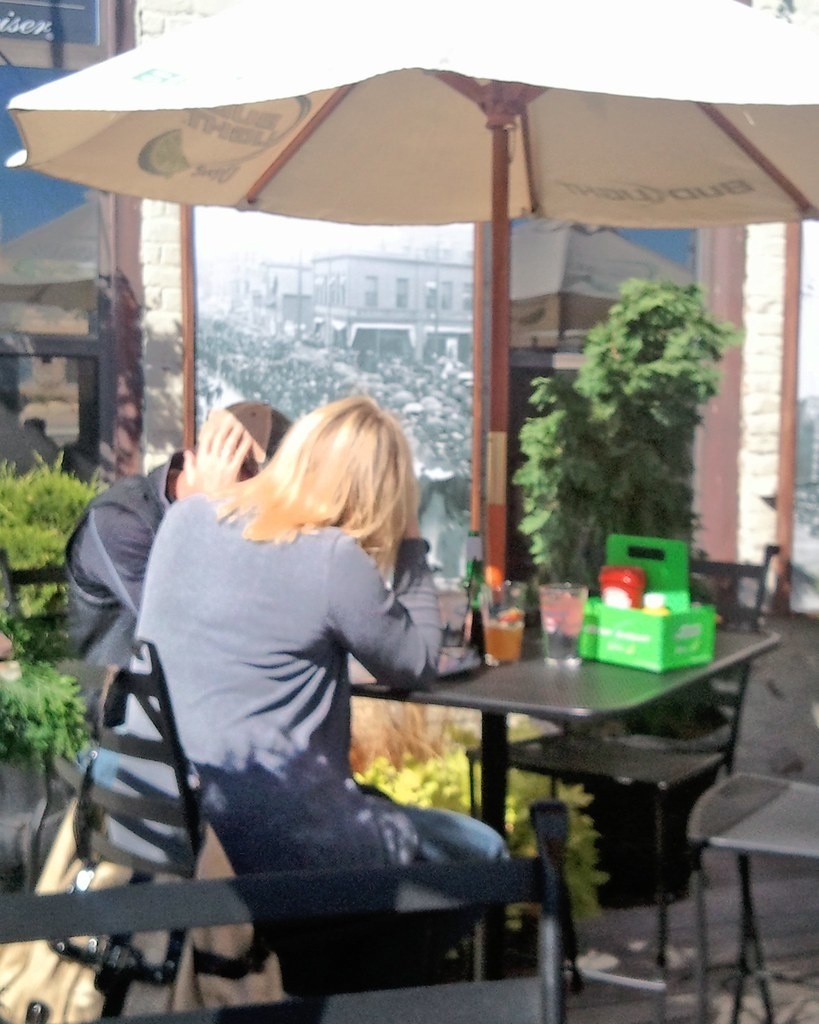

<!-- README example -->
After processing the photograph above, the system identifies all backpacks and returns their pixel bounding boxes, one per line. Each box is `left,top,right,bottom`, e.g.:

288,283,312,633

0,664,284,1024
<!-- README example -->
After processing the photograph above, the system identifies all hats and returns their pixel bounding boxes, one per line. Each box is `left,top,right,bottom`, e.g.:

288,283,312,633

198,401,293,477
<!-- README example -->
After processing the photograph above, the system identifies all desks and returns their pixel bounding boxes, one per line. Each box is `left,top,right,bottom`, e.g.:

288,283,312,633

340,629,780,1021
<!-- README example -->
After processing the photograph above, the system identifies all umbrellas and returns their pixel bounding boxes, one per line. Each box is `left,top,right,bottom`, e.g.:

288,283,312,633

0,194,97,331
507,221,696,350
6,0,818,589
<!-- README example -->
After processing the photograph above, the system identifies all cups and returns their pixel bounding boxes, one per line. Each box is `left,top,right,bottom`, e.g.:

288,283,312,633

539,583,589,663
479,580,526,662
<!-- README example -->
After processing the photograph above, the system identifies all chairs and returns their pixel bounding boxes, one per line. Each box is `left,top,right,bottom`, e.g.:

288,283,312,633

685,773,819,1024
1,640,492,1019
469,543,779,992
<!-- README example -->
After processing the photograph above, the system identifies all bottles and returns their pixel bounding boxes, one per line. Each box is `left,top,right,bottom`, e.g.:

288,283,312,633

639,591,669,616
455,530,489,658
599,564,646,609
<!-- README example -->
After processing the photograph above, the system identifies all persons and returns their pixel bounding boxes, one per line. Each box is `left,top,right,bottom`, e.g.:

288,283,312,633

65,401,295,731
128,395,514,994
